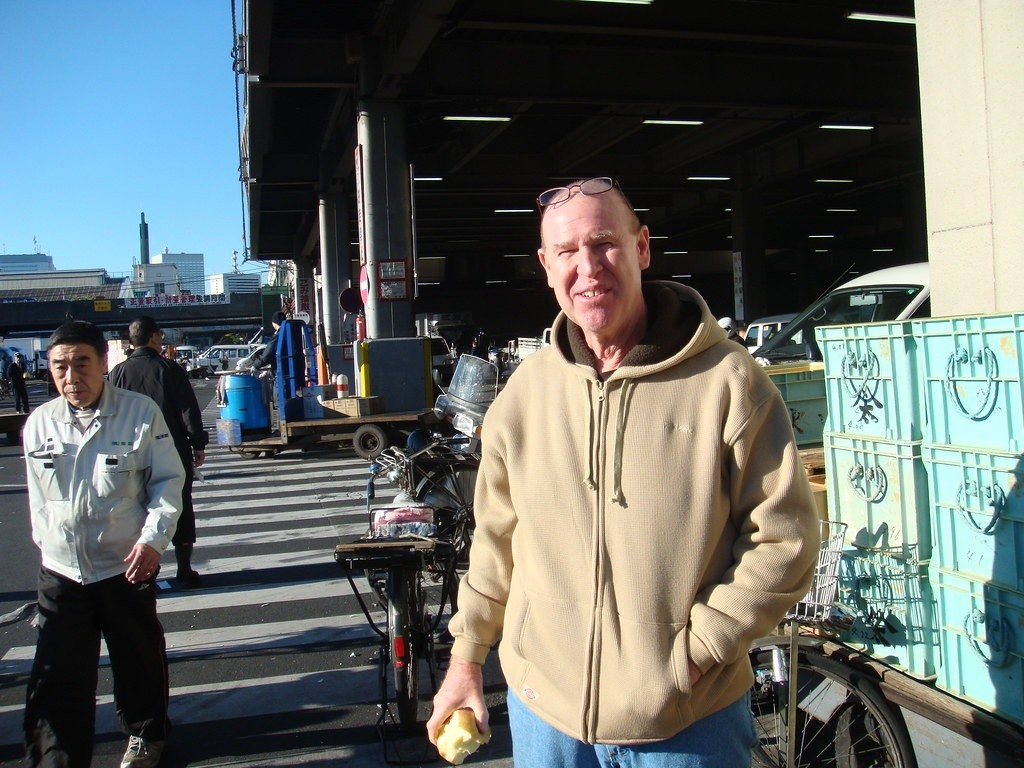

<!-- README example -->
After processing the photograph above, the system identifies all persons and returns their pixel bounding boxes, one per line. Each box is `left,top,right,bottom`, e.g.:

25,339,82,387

426,181,820,768
20,319,186,768
107,318,209,579
7,351,30,414
717,317,748,351
251,311,305,437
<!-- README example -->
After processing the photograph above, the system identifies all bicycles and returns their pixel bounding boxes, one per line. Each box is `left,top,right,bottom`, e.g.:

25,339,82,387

335,435,473,761
744,515,917,767
0,378,11,401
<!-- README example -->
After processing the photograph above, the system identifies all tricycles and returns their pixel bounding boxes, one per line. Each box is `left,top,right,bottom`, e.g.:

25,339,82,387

214,319,433,459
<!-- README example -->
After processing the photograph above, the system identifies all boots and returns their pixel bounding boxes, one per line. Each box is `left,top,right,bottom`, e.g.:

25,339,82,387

176,543,199,586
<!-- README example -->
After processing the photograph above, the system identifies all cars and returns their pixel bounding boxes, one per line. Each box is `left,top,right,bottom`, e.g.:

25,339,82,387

744,259,931,365
743,315,806,360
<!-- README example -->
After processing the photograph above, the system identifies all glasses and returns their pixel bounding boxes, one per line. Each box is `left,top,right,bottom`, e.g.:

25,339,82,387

536,177,634,218
158,330,165,340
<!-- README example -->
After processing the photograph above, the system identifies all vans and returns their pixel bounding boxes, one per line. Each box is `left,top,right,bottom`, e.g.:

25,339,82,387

197,344,271,371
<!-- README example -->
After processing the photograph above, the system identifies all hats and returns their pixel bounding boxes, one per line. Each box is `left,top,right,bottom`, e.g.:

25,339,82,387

272,311,285,325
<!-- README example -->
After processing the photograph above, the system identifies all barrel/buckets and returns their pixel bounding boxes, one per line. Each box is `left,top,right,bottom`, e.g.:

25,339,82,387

216,418,242,445
219,374,273,442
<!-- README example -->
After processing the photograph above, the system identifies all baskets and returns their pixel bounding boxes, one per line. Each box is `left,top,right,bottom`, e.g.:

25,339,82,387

781,519,847,622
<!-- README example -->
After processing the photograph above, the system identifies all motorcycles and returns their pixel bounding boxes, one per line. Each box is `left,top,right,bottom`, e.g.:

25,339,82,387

408,353,514,550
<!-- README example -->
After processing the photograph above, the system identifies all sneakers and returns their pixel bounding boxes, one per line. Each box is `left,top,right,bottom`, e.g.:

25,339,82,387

120,735,162,768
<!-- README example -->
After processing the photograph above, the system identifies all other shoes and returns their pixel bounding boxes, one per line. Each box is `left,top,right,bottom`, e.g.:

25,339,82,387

23,411,30,414
16,411,21,414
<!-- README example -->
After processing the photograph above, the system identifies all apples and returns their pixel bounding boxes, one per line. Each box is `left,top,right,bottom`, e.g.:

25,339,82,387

436,709,491,765
